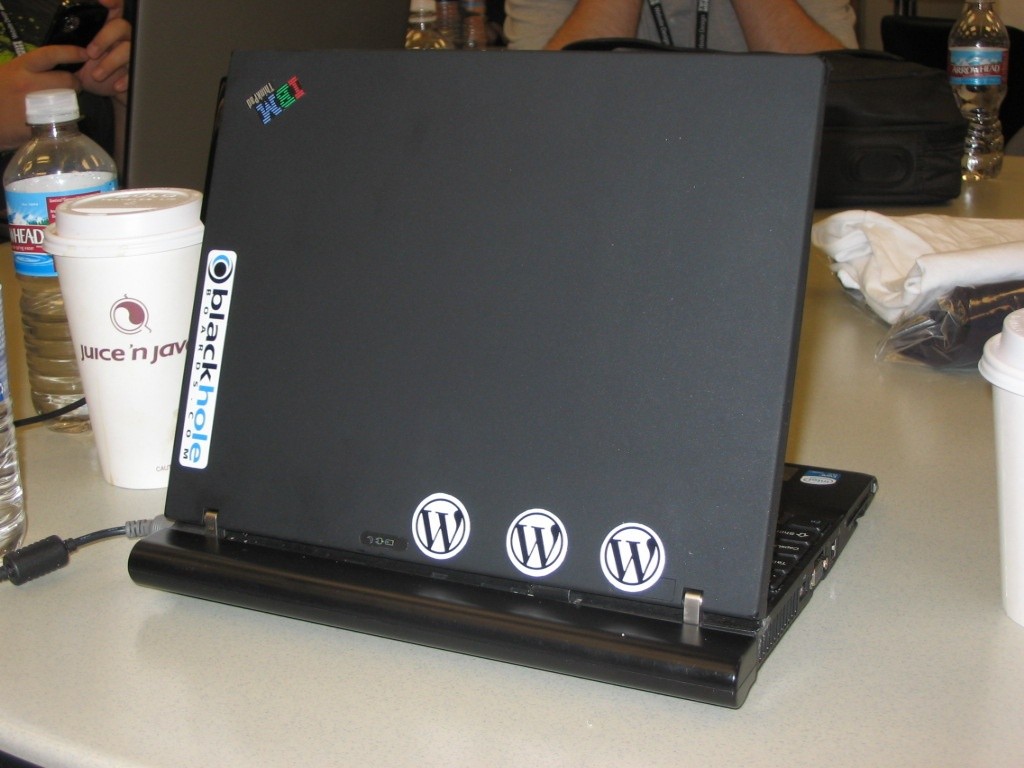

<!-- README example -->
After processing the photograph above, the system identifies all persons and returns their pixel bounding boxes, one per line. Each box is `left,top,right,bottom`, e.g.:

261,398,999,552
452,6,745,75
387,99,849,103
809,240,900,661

504,0,860,54
0,1,132,245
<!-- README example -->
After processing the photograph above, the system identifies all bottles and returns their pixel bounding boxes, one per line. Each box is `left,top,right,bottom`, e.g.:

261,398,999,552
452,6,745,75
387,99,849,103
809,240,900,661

942,0,1010,183
460,0,488,51
404,0,457,51
35,182,205,490
0,280,27,556
1,86,121,437
435,0,461,50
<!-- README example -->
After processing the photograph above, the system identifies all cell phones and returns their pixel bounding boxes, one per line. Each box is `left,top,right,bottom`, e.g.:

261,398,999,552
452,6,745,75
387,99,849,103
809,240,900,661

44,0,113,74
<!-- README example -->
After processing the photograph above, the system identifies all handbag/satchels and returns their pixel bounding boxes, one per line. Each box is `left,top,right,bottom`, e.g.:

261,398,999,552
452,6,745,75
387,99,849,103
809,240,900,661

562,38,967,206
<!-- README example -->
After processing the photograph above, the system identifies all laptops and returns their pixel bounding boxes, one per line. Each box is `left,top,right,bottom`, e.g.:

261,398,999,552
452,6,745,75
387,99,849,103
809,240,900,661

127,49,878,709
